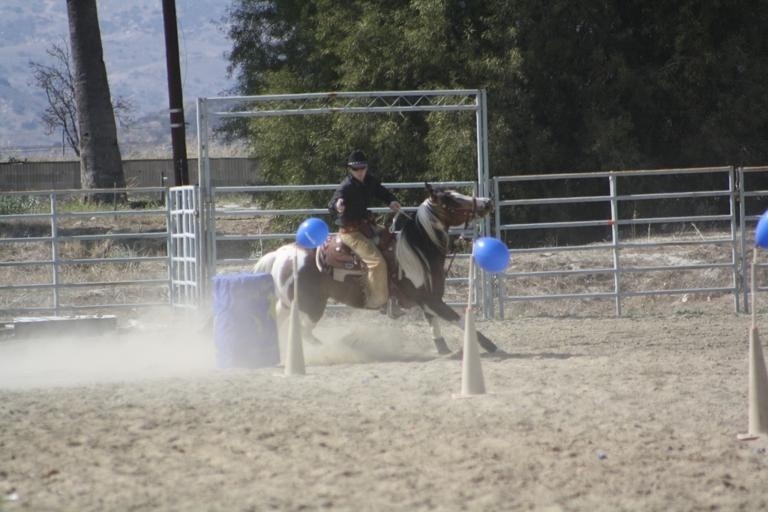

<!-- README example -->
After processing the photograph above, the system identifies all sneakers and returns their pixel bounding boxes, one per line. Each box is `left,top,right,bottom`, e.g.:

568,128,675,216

381,303,406,316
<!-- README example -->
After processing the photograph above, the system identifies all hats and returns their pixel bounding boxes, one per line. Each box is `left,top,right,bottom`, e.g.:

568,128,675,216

348,150,367,169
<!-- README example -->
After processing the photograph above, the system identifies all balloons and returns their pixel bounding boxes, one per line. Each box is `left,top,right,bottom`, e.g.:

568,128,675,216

473,236,510,274
295,217,329,249
755,211,768,251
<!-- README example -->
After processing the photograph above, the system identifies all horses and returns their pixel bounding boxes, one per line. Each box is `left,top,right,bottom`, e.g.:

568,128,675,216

251,179,511,359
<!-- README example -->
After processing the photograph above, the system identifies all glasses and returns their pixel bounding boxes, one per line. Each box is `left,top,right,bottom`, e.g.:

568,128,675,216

351,167,366,170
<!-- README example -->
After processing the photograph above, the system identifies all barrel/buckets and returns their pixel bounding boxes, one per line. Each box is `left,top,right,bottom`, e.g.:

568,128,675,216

213,273,281,368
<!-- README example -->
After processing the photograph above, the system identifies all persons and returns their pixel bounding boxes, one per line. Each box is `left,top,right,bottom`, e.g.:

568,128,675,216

327,150,407,319
